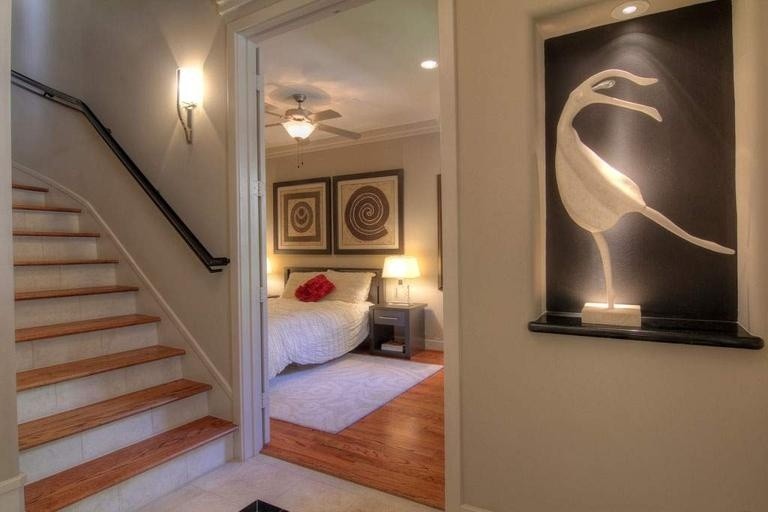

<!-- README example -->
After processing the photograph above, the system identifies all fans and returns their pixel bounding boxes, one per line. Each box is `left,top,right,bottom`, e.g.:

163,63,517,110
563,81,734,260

264,94,362,140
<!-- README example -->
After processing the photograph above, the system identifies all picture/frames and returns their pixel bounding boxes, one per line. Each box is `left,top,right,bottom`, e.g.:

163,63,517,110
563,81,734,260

273,177,331,255
436,174,443,289
332,169,404,255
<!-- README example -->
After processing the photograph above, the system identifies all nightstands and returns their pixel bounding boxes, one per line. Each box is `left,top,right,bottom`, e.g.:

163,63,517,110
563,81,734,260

368,302,427,359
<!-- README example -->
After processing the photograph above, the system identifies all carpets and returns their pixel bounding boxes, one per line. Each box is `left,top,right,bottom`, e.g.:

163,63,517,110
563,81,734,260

270,354,444,435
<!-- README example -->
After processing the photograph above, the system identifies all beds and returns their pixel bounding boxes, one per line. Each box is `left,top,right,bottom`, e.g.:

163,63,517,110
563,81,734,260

267,266,386,381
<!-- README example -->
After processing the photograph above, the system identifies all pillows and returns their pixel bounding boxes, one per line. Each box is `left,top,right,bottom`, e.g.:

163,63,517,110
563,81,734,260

326,270,376,304
280,272,326,298
295,274,336,301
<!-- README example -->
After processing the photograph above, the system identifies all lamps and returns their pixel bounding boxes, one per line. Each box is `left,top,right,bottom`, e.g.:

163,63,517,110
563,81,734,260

176,67,204,144
282,94,317,141
382,255,421,304
611,0,650,20
